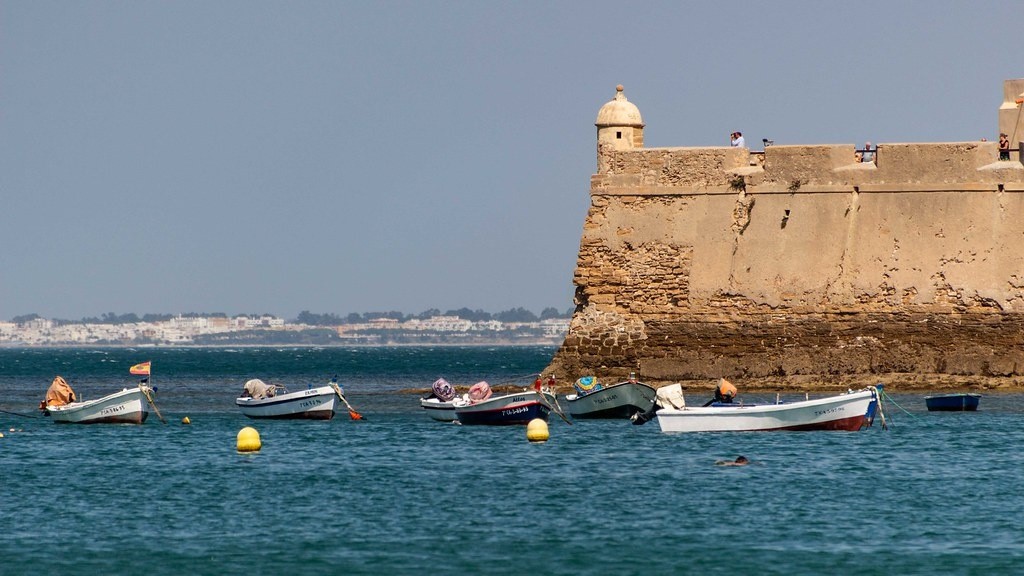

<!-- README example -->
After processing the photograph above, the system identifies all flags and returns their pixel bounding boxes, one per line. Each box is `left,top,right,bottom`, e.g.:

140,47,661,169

130,362,150,375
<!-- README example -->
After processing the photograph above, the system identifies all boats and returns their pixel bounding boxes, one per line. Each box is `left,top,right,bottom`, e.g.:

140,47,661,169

39,360,158,424
235,374,345,420
419,377,469,422
631,379,884,433
453,373,557,425
566,372,656,419
925,393,981,411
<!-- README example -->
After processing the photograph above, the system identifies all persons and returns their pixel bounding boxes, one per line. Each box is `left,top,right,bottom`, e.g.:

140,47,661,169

724,456,748,465
998,133,1010,161
730,132,744,147
982,138,986,142
548,376,555,392
535,377,542,393
860,143,873,162
